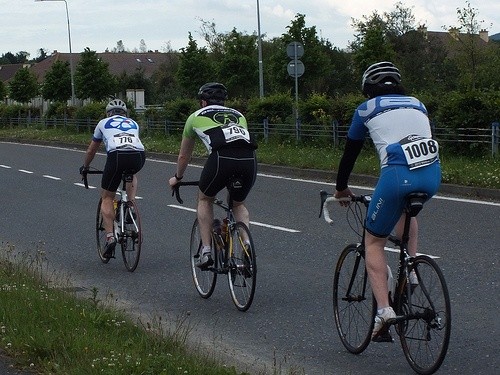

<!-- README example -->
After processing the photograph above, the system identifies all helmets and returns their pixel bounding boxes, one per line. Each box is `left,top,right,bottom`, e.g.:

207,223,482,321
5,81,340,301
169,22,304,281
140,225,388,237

361,61,401,98
105,99,128,113
196,82,228,103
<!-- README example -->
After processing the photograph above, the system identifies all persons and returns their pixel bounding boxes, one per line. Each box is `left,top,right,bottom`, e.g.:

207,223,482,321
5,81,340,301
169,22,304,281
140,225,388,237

168,81,259,268
78,99,147,259
334,59,442,341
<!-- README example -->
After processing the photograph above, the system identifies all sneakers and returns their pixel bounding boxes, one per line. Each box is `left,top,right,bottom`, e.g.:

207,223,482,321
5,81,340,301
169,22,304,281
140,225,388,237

102,236,116,258
371,307,397,341
405,269,419,296
239,240,252,265
125,206,137,224
195,252,213,268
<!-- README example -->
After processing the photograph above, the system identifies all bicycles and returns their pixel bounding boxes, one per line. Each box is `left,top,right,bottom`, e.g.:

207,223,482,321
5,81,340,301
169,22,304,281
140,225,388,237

170,182,257,312
79,164,144,272
317,191,452,375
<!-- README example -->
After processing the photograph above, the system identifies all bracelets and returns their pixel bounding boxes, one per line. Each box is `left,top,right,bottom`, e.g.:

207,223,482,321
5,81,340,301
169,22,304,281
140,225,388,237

175,173,183,180
